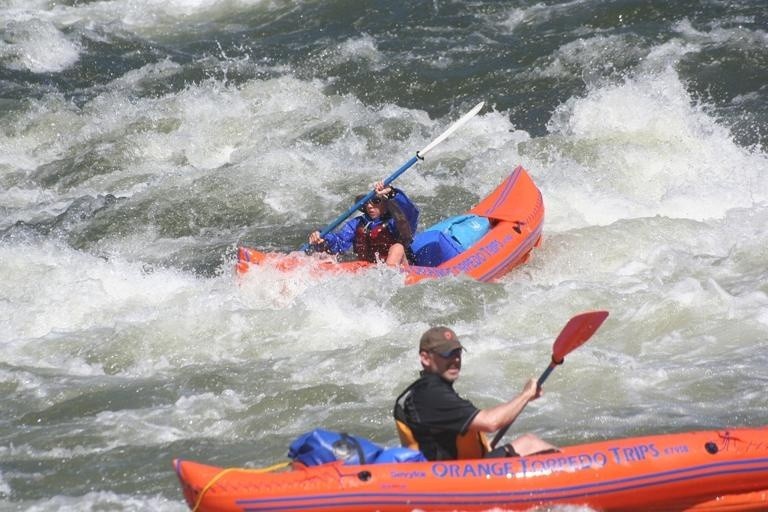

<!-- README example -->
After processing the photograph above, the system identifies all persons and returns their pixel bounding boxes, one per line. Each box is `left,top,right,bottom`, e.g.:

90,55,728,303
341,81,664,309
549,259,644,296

392,326,556,462
307,181,414,265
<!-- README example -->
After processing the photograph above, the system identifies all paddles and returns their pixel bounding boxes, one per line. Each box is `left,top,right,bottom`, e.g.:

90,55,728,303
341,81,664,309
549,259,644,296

296,100,485,251
490,311,609,449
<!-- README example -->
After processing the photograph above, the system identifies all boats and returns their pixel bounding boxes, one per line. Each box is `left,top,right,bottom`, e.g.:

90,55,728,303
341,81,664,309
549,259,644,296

168,424,768,512
230,158,550,291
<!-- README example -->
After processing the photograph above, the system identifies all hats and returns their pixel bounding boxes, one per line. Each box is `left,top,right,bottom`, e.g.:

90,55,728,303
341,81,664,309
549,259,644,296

419,327,467,357
356,182,384,210
